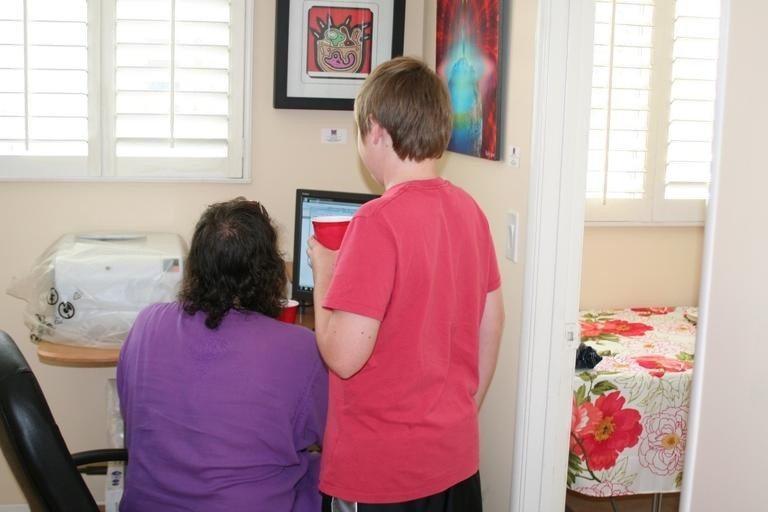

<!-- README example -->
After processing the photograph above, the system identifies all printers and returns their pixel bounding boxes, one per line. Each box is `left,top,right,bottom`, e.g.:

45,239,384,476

50,227,188,343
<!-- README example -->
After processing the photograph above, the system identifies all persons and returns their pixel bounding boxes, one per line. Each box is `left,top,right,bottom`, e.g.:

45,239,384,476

305,53,504,512
114,195,330,512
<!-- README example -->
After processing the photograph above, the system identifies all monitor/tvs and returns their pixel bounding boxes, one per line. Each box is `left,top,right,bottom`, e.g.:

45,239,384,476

290,185,383,305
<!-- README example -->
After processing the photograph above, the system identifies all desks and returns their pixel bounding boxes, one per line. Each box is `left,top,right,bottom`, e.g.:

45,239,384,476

569,307,698,511
37,302,325,510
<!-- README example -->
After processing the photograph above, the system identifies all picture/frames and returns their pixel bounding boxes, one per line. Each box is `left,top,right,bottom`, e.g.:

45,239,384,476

272,1,405,111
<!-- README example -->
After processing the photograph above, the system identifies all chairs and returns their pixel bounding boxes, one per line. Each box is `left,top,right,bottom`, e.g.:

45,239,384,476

0,325,129,510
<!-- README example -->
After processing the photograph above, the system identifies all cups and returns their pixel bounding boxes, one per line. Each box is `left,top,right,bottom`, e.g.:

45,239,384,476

307,216,354,251
272,298,301,325
